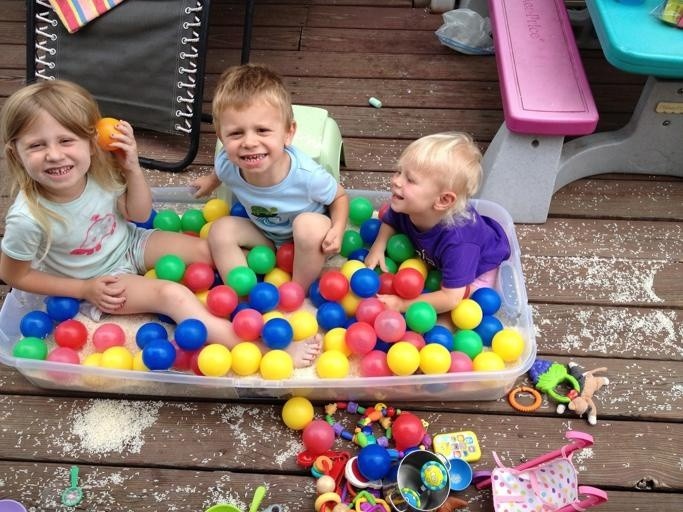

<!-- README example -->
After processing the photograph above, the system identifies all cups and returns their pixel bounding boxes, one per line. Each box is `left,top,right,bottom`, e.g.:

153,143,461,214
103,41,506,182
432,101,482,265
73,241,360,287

381,449,451,512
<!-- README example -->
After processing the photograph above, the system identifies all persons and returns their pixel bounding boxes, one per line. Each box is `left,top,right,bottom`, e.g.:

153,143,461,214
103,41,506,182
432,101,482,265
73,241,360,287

0,80,321,367
363,131,511,334
191,64,348,304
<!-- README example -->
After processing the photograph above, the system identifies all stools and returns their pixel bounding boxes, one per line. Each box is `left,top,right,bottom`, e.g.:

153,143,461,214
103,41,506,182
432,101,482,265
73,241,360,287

214,106,347,202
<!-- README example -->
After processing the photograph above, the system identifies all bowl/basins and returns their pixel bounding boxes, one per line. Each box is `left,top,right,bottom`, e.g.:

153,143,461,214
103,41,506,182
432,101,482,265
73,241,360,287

444,459,473,492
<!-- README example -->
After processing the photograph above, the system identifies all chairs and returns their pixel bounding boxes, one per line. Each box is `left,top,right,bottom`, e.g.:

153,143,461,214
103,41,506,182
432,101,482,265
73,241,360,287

25,0,256,171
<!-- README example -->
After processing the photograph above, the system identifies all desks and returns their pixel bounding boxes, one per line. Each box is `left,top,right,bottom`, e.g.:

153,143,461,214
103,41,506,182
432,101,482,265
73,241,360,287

551,0,683,198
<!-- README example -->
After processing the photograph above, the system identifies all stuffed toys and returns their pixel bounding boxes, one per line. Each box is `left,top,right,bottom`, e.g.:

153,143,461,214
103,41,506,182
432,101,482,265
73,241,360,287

557,365,612,426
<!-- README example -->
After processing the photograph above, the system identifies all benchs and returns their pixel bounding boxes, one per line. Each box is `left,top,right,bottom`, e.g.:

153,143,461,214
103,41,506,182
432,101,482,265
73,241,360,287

472,0,599,225
